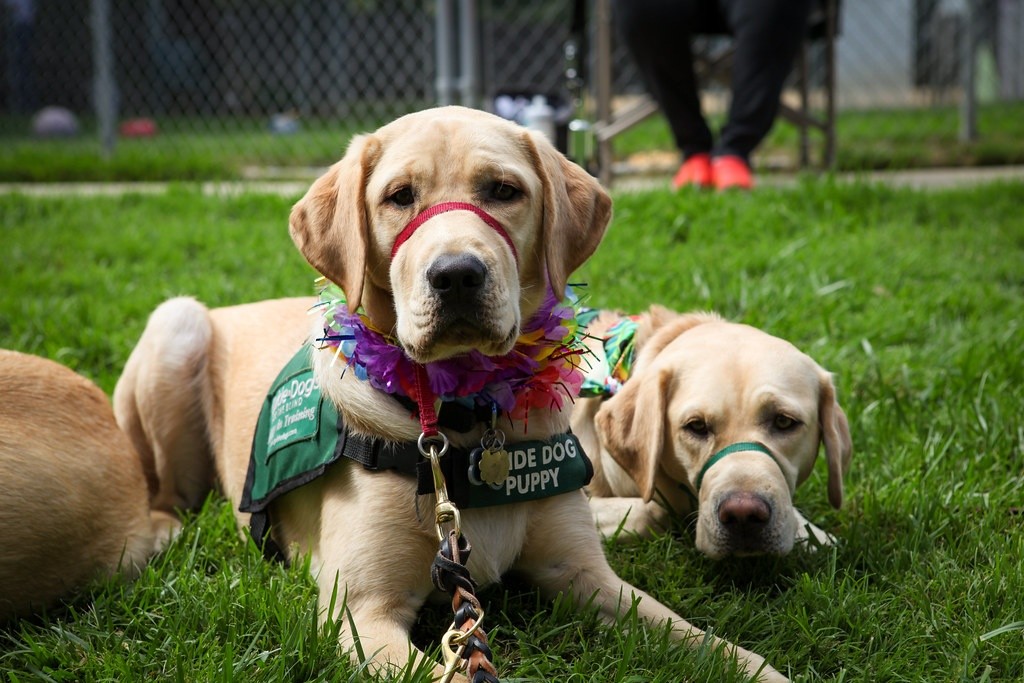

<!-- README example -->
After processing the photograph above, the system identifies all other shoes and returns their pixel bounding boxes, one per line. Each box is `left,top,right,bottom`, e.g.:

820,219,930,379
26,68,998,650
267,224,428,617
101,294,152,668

709,155,752,189
671,154,710,188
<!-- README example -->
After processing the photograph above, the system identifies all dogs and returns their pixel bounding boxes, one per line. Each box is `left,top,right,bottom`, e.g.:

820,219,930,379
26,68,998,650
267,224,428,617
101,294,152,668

567,303,852,564
0,347,183,627
113,105,792,683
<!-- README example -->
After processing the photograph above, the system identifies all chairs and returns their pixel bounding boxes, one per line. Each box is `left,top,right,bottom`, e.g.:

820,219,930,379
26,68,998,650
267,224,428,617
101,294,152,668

586,0,836,190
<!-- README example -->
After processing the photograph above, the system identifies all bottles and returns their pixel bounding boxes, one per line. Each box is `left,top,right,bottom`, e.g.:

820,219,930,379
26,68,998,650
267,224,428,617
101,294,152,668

564,119,600,179
522,94,555,145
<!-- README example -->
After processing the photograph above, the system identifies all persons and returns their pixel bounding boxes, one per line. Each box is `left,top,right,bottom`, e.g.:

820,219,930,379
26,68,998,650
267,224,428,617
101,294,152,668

616,0,819,189
